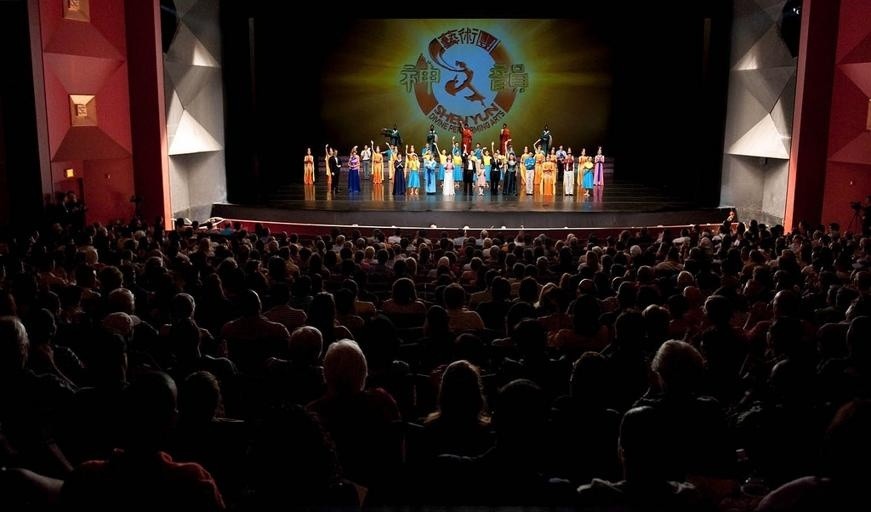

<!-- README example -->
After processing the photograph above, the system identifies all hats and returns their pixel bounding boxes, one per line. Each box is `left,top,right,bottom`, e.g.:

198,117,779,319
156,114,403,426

145,256,163,269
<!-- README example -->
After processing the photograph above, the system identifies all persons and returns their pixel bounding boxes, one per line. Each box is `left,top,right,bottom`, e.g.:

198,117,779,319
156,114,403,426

303,121,604,195
1,190,870,511
